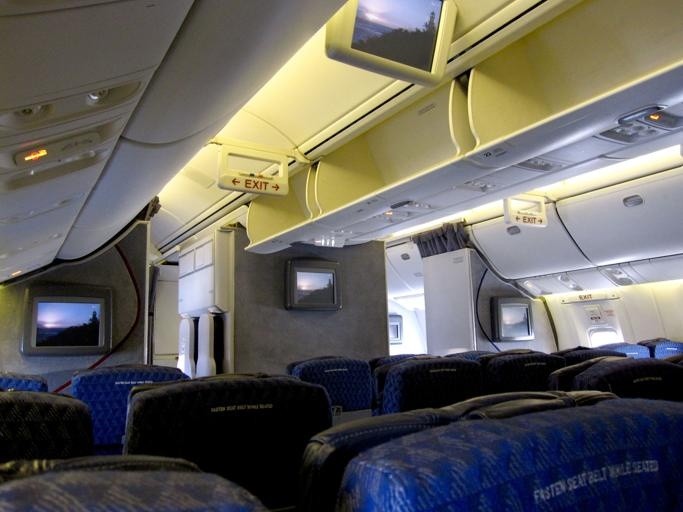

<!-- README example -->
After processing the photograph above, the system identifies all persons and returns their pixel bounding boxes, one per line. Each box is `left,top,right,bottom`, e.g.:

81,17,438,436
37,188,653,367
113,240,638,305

89,310,98,323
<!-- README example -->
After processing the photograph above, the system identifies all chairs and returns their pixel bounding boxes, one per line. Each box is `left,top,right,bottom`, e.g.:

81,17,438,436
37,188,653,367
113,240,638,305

1,338,683,512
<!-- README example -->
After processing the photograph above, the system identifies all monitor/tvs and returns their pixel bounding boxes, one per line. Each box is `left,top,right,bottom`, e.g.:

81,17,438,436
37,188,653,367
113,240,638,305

388,314,404,345
490,296,536,343
324,1,459,89
285,258,343,312
20,282,116,358
